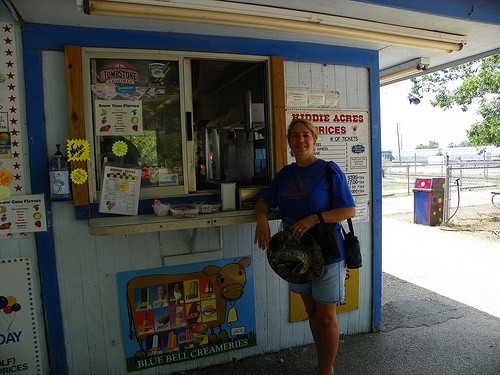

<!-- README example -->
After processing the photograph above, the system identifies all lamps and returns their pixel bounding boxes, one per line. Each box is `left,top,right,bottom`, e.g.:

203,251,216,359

76,0,468,53
198,159,206,178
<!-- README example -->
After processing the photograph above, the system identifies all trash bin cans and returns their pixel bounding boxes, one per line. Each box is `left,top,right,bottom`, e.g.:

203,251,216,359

412,178,446,226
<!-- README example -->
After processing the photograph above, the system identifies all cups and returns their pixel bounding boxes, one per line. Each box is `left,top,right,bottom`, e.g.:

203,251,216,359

139,280,216,330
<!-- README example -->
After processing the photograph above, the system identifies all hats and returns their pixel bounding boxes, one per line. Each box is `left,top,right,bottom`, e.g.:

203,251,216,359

267,230,325,285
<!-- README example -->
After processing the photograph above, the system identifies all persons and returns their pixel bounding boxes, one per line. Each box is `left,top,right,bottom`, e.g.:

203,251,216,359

253,119,356,375
102,136,153,185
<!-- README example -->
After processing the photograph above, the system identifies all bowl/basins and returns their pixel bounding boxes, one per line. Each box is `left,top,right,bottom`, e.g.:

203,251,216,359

152,202,171,217
169,203,200,219
192,201,222,213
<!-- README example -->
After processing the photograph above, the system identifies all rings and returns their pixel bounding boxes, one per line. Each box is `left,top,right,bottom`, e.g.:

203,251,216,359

298,228,301,232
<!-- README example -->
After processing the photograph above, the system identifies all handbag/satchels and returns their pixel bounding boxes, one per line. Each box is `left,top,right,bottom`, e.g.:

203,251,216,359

343,231,362,269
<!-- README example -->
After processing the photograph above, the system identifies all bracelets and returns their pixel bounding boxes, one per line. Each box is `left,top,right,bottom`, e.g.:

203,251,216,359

316,212,325,223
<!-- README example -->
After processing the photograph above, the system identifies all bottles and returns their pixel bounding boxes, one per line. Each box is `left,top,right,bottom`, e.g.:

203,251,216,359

47,144,72,199
